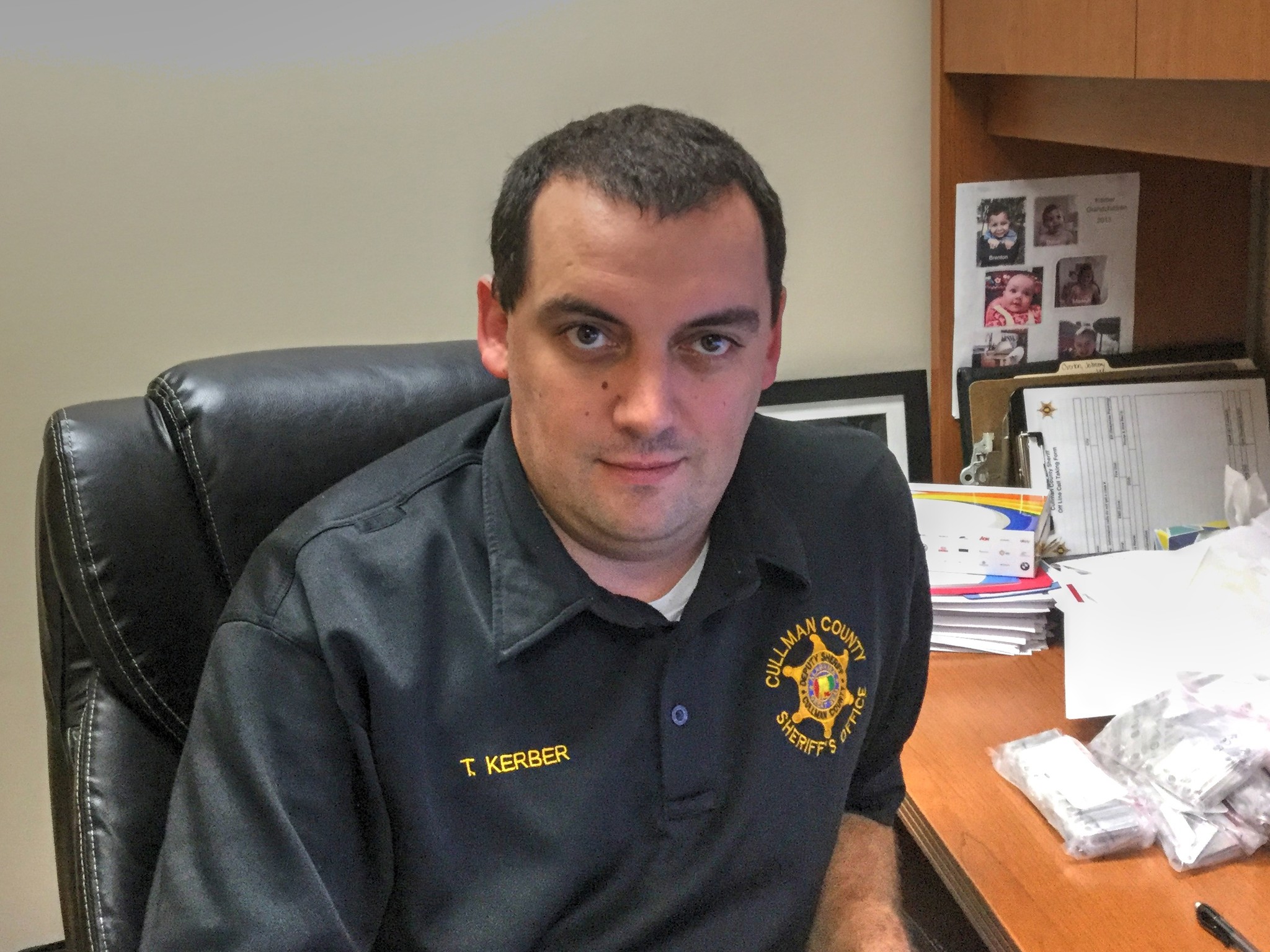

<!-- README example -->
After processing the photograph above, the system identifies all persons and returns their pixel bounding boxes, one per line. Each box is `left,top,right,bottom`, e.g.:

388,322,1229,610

137,105,933,952
974,203,1107,368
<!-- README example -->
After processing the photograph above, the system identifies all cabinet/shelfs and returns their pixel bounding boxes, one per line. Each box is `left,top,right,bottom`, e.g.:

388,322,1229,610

931,0,1270,81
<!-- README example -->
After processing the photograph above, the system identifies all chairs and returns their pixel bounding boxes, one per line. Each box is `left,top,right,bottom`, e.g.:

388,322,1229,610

35,340,511,952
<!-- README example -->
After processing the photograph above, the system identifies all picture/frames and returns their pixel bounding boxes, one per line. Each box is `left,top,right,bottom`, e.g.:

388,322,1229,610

753,369,934,483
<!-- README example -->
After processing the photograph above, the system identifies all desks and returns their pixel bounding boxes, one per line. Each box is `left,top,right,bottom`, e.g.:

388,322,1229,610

899,637,1270,952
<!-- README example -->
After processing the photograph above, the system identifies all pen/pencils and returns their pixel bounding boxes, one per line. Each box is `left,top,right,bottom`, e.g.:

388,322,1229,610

1193,903,1258,951
